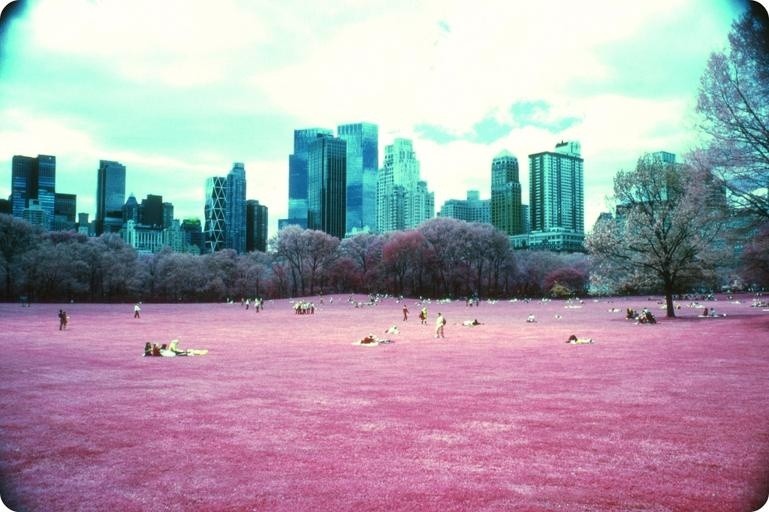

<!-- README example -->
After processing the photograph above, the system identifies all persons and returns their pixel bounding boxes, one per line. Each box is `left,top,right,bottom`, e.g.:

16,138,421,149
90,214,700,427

260,297,264,310
402,305,410,321
346,291,404,309
153,343,160,356
330,297,333,303
416,294,452,305
662,289,767,319
567,334,592,344
161,343,167,350
169,339,187,356
461,318,482,326
294,299,315,315
58,309,64,330
245,298,250,309
62,312,67,326
133,303,141,318
486,291,551,306
254,298,260,312
419,306,428,324
464,296,469,307
475,295,480,307
608,307,622,313
469,296,474,307
626,306,658,325
144,342,152,355
526,313,535,323
434,312,445,338
